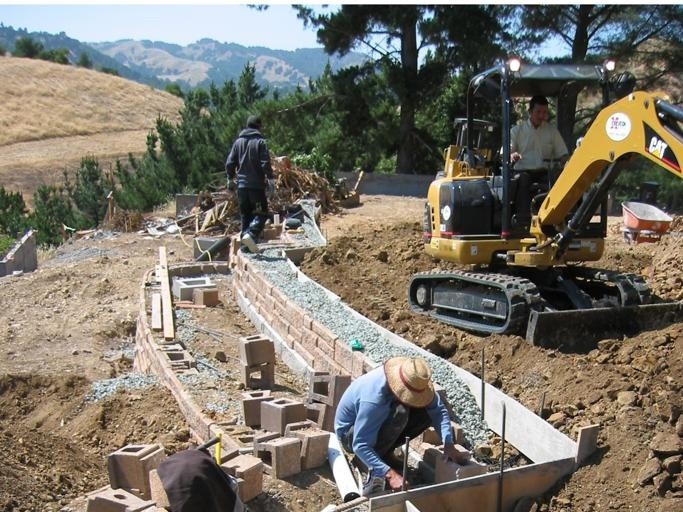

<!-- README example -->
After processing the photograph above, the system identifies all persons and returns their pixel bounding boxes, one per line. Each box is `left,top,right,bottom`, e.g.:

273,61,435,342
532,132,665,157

498,95,572,233
223,113,273,255
332,356,467,492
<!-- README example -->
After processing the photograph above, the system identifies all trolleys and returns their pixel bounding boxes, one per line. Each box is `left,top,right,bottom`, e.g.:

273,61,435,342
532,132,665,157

620,201,673,243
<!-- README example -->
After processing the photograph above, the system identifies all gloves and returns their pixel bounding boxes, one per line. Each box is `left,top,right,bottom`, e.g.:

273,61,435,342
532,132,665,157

227,180,236,191
268,178,275,186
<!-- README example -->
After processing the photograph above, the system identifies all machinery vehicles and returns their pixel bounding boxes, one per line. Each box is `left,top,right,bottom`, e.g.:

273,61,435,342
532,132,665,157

406,57,682,345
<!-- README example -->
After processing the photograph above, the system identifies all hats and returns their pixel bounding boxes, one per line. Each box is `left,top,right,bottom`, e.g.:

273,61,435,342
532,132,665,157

384,355,436,408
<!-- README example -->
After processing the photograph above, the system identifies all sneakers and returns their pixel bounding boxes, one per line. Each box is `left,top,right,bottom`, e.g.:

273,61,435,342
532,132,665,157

241,233,259,253
